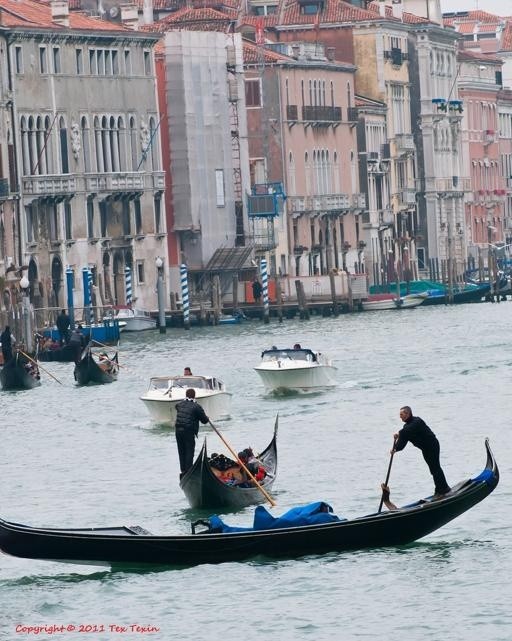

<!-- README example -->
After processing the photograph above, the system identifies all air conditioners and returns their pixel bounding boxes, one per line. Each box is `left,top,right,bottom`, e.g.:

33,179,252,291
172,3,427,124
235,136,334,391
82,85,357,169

368,151,378,160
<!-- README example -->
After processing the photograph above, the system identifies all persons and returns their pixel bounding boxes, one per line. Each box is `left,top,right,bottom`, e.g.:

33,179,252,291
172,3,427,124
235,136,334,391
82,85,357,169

174,389,209,474
251,278,262,302
184,367,192,375
0,309,113,381
293,344,301,349
390,406,451,502
207,448,265,488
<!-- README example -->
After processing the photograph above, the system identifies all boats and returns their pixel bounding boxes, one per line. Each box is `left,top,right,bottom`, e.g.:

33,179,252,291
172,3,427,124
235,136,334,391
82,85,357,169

0,336,45,389
178,411,281,510
74,336,121,384
362,254,512,313
251,347,339,393
35,306,241,362
0,437,500,563
141,371,232,431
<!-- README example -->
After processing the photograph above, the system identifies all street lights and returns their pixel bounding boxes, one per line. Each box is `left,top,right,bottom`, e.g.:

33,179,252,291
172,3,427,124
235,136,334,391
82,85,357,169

20,277,33,353
389,246,409,299
152,256,168,330
